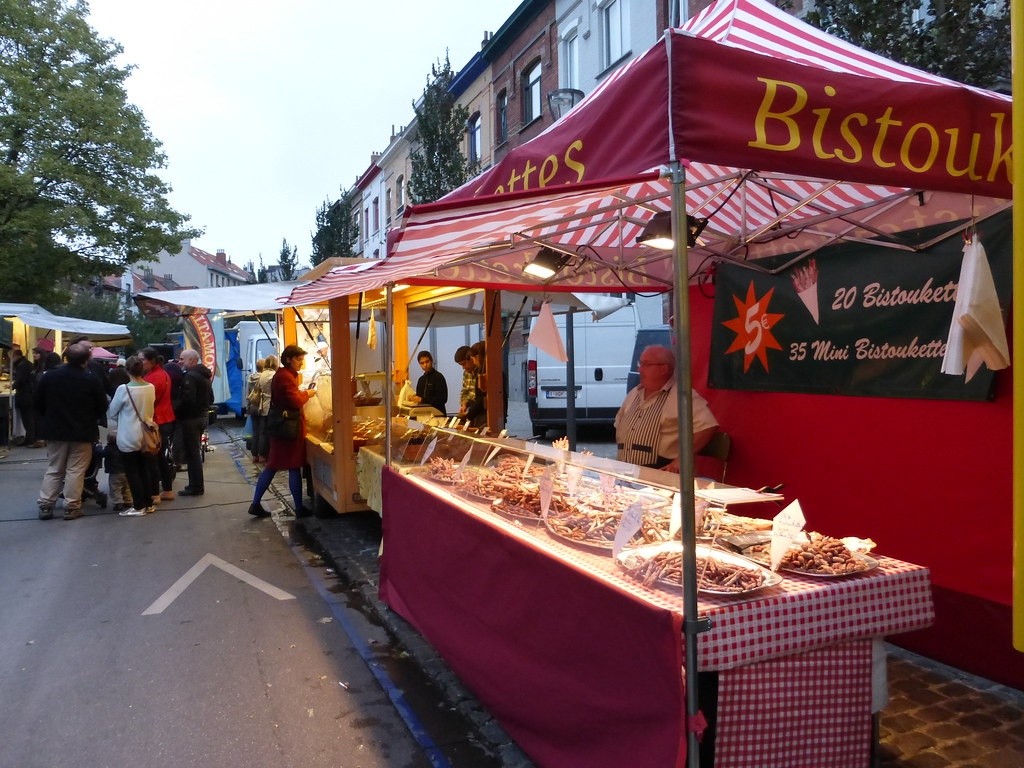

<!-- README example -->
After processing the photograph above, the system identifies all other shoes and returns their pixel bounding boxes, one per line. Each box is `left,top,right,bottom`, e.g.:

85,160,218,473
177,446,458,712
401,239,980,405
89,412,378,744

26,439,47,449
259,458,267,463
118,507,146,517
18,438,32,446
178,490,205,496
159,490,175,501
184,485,190,489
247,503,272,518
64,507,85,520
125,503,132,508
150,495,161,505
252,456,258,463
112,504,124,512
38,504,54,520
145,505,156,513
296,505,312,518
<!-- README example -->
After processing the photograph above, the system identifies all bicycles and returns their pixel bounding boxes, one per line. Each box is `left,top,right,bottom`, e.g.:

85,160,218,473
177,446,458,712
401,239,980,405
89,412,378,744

200,409,215,464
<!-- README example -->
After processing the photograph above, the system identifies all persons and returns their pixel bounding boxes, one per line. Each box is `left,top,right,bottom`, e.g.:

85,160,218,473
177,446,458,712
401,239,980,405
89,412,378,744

248,345,317,517
4,341,215,519
248,354,278,462
613,345,720,473
411,351,448,417
454,341,508,429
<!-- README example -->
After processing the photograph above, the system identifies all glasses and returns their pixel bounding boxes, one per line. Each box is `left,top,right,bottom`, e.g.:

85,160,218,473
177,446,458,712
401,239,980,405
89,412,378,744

637,361,672,370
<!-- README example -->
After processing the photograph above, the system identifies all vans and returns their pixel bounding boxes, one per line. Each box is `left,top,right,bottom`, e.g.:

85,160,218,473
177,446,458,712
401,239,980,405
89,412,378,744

527,301,642,440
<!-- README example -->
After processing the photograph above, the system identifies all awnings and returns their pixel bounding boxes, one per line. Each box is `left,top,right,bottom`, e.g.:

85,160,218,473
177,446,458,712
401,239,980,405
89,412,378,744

131,279,331,369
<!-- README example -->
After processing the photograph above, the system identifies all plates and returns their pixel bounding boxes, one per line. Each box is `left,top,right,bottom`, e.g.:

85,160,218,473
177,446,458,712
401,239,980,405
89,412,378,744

424,440,884,598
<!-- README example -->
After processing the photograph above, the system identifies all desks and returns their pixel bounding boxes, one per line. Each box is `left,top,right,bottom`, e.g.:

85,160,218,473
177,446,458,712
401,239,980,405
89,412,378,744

356,442,934,768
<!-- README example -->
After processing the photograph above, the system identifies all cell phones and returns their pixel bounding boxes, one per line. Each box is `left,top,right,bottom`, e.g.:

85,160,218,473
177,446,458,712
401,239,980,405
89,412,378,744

308,383,316,389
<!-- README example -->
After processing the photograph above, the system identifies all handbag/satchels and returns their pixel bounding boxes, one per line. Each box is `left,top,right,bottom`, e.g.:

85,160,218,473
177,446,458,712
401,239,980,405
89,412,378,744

264,402,301,438
246,389,261,407
240,413,254,442
141,420,161,455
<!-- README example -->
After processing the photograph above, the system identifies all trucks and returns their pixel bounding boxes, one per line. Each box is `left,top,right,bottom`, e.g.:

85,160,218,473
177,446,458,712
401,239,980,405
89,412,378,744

233,320,278,423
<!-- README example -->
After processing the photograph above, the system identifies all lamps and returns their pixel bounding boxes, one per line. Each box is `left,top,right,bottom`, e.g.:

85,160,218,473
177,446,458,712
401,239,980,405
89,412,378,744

636,211,708,251
522,247,571,280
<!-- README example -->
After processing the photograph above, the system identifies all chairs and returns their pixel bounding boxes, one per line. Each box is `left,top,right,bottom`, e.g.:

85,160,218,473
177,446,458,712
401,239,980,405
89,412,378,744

696,432,731,482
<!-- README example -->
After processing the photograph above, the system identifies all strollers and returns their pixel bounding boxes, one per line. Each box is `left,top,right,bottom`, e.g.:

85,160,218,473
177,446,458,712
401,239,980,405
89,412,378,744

62,441,107,509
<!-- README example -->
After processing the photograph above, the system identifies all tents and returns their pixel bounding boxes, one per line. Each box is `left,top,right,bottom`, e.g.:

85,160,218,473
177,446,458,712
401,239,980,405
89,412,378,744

0,303,134,446
281,1,1013,768
